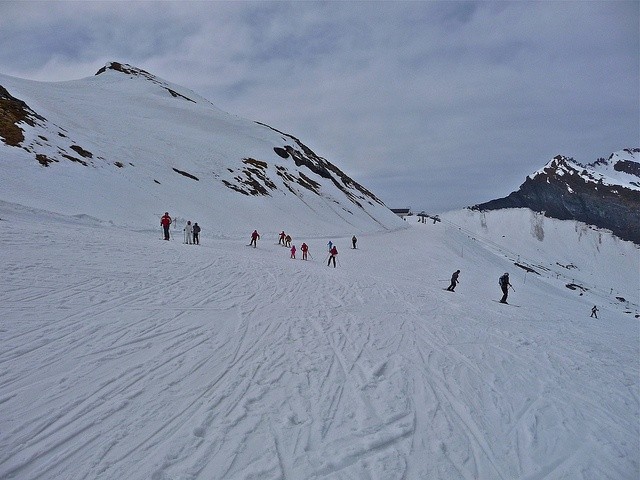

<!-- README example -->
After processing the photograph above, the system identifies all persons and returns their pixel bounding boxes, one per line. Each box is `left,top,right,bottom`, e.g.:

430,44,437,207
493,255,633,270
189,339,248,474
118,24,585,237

289,245,296,259
589,306,599,318
278,230,286,245
327,241,333,252
447,269,460,292
160,212,172,240
498,273,515,305
183,220,193,245
352,235,357,249
192,222,201,245
284,234,292,248
300,242,308,261
249,230,260,248
327,245,338,268
